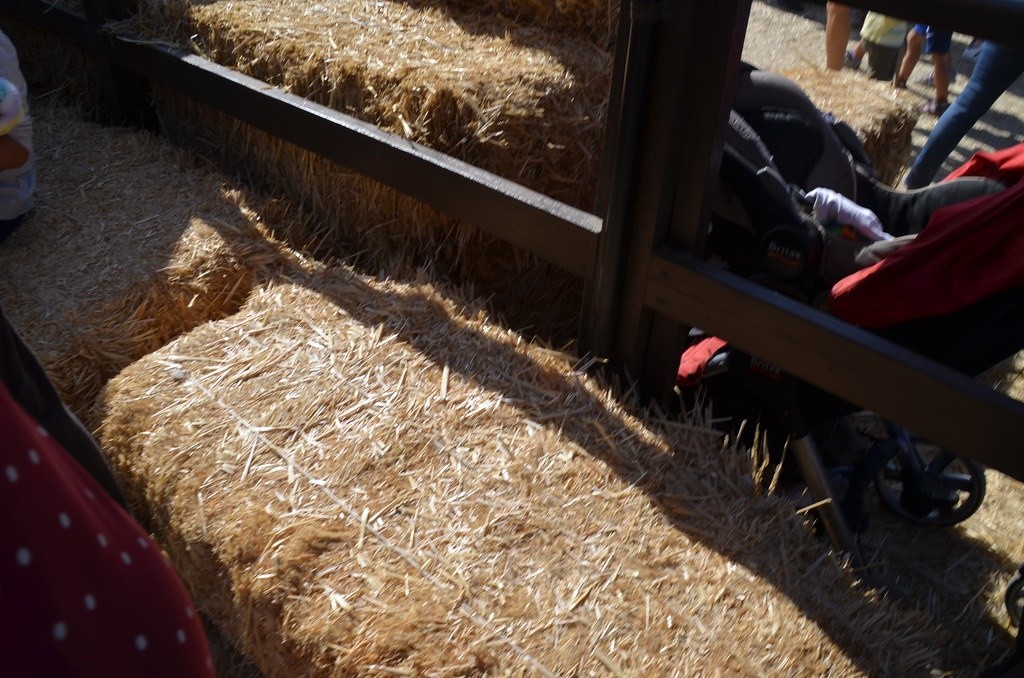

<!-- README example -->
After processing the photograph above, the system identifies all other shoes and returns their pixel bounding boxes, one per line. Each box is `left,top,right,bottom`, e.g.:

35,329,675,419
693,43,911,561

923,98,949,114
846,50,862,69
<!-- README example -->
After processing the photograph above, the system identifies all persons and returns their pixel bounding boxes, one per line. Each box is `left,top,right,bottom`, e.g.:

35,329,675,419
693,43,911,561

0,31,36,246
824,0,1024,190
